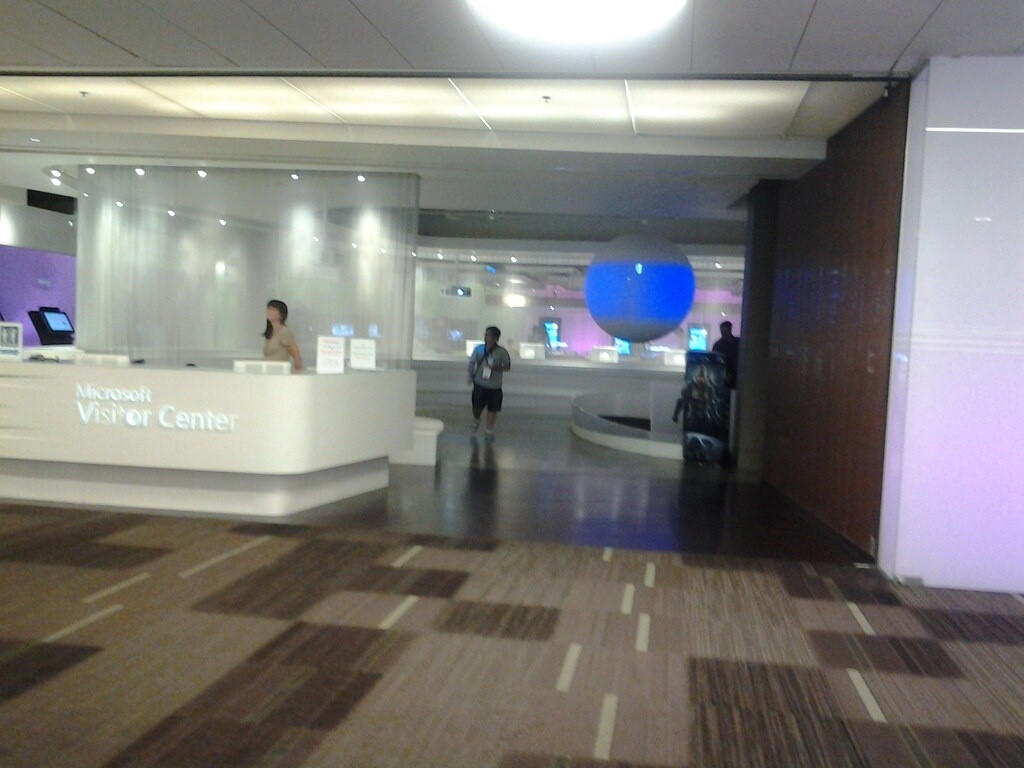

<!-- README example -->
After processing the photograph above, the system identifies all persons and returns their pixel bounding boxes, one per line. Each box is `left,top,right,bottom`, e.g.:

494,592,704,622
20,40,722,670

467,327,511,441
263,300,302,371
712,321,740,376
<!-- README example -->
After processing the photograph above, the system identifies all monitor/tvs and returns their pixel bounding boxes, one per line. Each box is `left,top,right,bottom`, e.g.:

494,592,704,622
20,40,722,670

40,310,75,334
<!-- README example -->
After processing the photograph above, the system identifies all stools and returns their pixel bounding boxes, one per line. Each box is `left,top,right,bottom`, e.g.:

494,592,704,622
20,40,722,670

389,417,444,466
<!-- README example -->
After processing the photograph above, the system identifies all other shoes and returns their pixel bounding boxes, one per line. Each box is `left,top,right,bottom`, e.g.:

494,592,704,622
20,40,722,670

485,430,495,441
471,418,481,432
